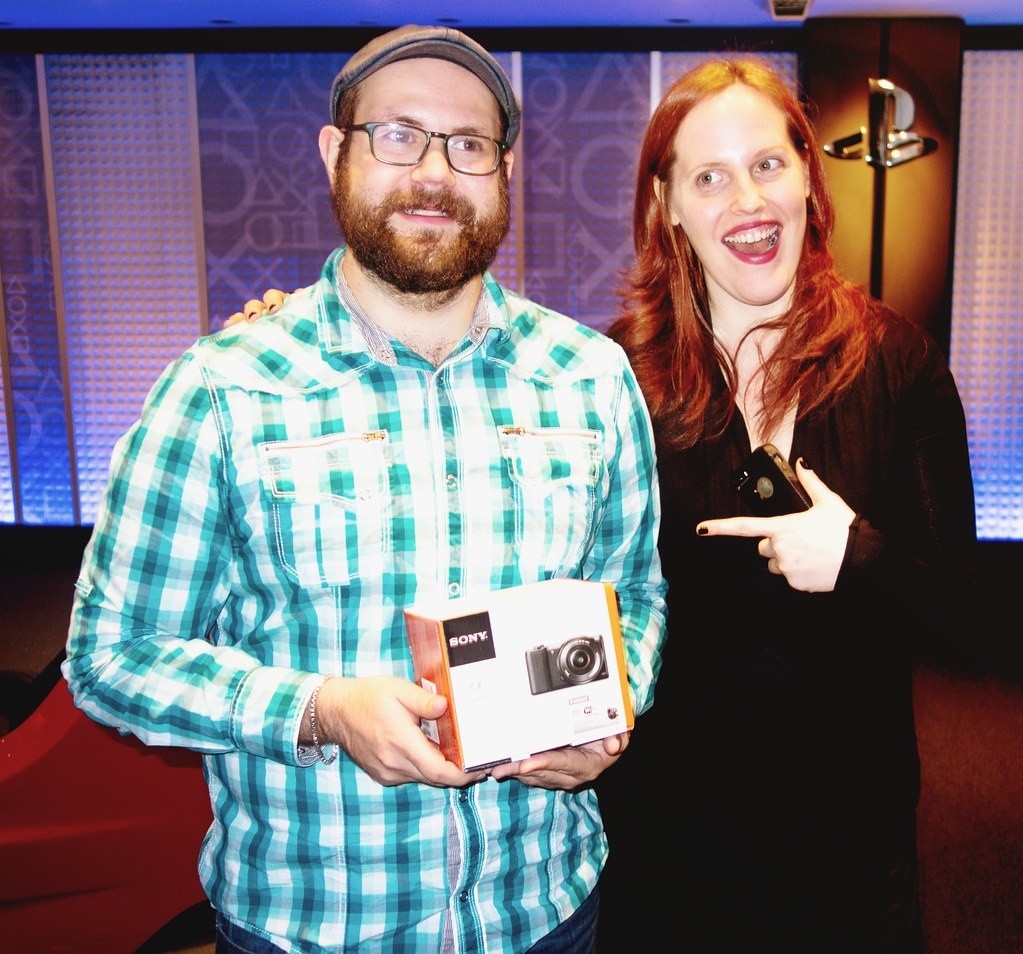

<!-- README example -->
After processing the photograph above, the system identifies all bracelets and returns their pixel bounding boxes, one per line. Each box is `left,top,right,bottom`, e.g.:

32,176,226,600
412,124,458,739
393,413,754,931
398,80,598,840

311,677,341,765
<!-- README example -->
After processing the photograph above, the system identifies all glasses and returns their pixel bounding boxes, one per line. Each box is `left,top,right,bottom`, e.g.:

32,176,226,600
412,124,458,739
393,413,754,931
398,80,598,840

341,121,509,175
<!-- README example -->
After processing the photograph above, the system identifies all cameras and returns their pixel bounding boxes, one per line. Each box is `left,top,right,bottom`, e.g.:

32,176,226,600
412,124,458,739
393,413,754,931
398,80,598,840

523,635,608,696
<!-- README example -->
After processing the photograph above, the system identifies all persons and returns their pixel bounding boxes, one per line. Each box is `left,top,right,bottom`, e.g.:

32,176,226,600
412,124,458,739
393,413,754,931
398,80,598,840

54,21,677,954
216,50,986,953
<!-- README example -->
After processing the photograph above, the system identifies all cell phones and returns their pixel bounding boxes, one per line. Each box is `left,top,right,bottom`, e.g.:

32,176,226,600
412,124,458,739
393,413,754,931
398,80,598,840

735,443,814,522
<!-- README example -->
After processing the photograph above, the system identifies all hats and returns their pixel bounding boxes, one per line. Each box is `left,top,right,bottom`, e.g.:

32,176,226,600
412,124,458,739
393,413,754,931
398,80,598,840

329,23,524,149
